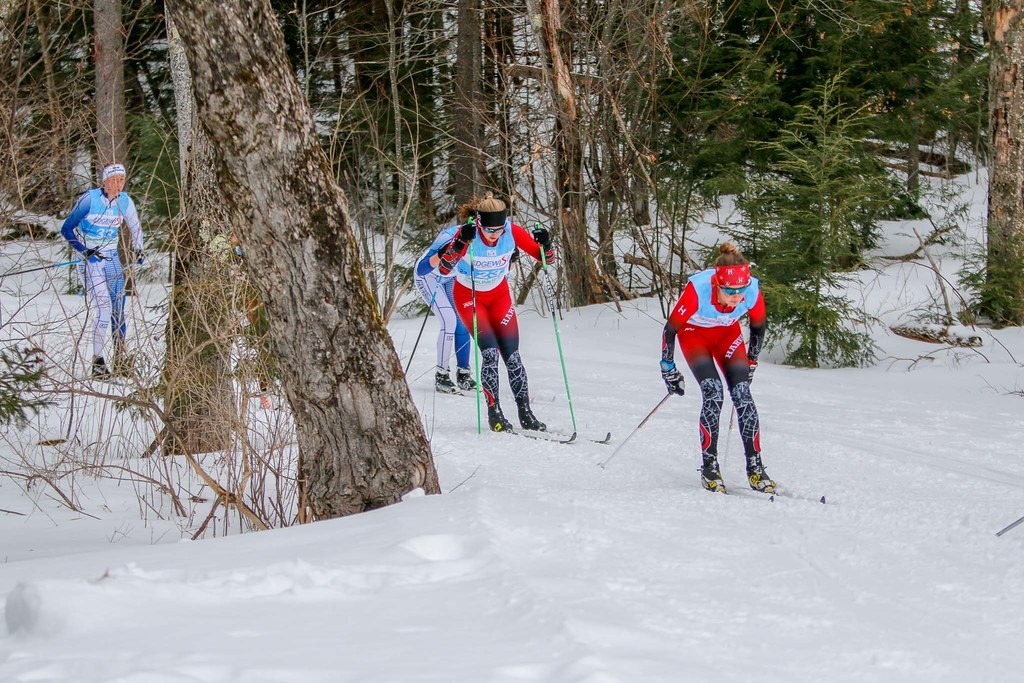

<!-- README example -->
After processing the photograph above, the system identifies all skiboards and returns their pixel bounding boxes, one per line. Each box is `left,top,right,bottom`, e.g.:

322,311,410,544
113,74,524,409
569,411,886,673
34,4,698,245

424,383,556,406
502,425,612,445
719,482,828,505
79,360,162,390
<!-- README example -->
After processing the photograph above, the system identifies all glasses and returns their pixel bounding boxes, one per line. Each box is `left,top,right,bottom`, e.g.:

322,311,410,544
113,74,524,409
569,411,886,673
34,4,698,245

109,177,125,182
717,278,751,296
481,223,508,233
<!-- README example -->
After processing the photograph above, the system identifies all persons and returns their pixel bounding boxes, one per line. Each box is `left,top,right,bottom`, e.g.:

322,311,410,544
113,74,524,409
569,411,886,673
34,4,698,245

230,233,270,409
60,159,146,382
659,243,778,495
413,196,520,396
438,191,555,432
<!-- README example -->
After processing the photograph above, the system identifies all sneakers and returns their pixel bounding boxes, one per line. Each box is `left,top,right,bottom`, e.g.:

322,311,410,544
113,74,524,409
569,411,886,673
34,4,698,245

516,397,547,430
92,365,113,383
434,365,460,394
455,365,484,392
696,465,727,494
487,404,513,432
746,469,776,493
114,363,133,377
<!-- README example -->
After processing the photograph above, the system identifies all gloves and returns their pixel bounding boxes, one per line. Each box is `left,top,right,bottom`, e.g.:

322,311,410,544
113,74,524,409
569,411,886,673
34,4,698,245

452,222,476,253
746,358,758,390
437,241,452,259
134,250,145,264
532,228,552,252
660,361,684,396
84,246,103,265
